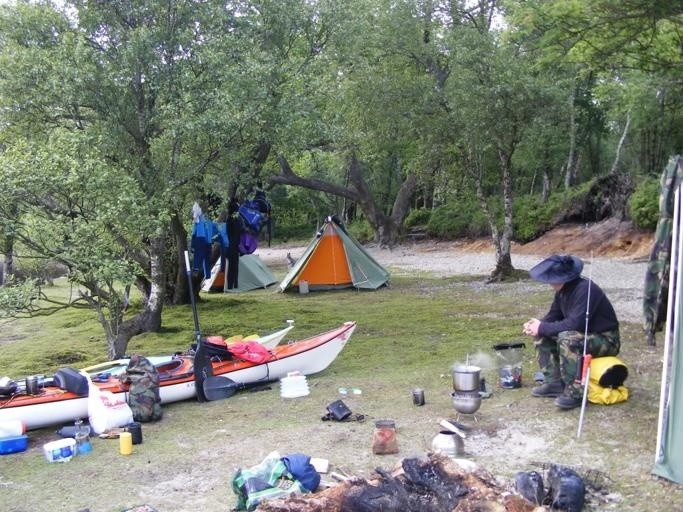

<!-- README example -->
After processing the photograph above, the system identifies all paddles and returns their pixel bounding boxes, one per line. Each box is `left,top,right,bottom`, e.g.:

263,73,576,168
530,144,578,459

184,252,213,401
203,376,279,399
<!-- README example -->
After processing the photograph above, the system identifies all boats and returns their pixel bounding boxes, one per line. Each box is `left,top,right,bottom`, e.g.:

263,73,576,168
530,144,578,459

0,321,357,441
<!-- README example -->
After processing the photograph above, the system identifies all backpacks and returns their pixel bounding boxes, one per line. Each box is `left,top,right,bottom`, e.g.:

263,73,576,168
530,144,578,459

119,354,164,423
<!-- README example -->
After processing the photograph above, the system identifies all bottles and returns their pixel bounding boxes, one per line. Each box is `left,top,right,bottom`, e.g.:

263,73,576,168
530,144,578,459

55,425,93,439
431,431,464,459
412,389,424,406
372,420,398,453
73,417,92,455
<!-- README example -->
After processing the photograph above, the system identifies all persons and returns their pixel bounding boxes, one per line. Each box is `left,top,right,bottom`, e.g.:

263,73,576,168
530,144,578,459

520,254,620,411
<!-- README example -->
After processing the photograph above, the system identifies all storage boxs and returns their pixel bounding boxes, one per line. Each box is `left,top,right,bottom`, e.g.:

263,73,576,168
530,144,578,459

1,434,30,455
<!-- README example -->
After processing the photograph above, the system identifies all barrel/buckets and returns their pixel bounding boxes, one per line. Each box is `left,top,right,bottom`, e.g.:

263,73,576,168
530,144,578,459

280,372,310,399
124,423,142,446
300,279,309,294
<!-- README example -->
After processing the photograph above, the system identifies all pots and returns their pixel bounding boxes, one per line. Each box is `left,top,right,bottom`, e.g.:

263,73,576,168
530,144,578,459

451,366,481,393
449,391,481,414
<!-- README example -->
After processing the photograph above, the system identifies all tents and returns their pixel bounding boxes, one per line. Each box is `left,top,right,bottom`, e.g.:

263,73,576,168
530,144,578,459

273,212,391,295
199,244,277,292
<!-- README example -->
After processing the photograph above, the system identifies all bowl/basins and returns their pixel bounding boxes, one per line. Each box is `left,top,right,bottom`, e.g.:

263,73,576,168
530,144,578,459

0,435,28,455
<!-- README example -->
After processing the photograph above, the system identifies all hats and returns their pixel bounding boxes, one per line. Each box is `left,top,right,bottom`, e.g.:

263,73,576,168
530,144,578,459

527,253,584,285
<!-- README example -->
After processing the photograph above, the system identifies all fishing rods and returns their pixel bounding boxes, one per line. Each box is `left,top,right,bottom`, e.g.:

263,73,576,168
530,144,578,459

576,251,592,440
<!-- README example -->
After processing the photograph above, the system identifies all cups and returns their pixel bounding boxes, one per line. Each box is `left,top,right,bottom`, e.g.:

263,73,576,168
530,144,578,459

123,422,142,444
496,338,524,390
119,432,132,455
25,375,38,396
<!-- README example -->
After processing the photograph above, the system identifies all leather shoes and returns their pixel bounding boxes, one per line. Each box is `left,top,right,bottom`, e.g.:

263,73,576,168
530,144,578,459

530,381,566,398
554,384,583,410
